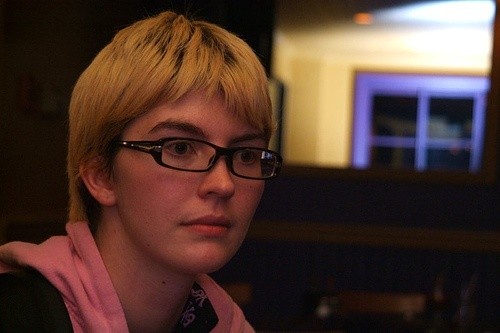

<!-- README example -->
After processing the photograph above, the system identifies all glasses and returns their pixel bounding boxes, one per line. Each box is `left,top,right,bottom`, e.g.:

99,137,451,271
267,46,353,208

113,137,283,181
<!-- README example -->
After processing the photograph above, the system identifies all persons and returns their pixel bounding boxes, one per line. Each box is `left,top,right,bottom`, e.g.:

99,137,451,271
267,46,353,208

0,8,276,333
